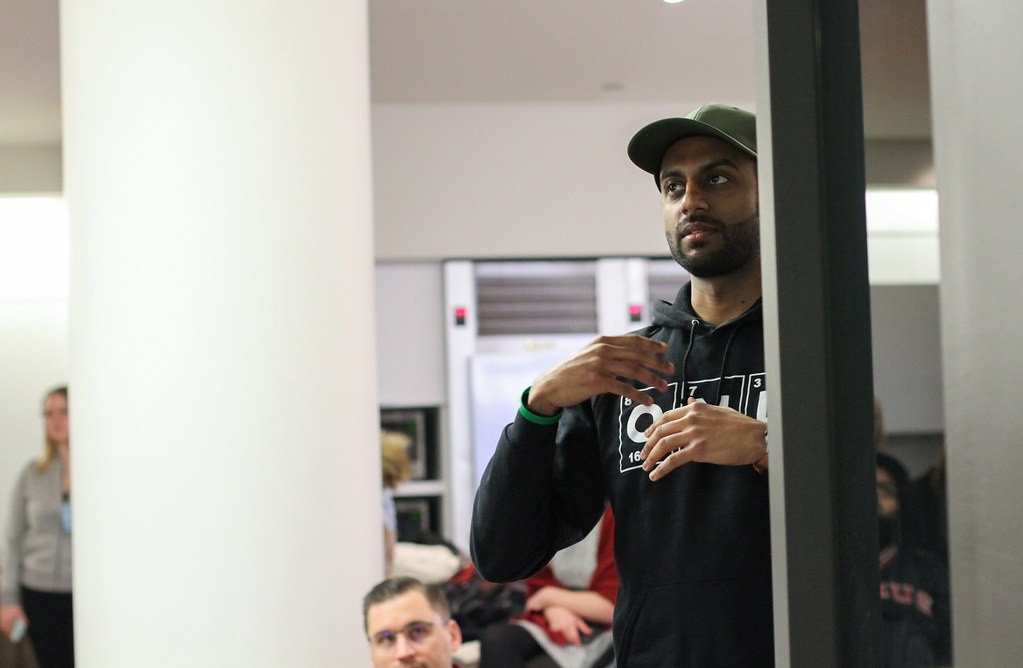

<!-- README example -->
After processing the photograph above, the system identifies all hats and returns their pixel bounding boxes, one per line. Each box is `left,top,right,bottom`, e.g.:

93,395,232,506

628,104,757,191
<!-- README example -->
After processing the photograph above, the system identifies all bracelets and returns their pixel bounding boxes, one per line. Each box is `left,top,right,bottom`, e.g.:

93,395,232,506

519,387,563,426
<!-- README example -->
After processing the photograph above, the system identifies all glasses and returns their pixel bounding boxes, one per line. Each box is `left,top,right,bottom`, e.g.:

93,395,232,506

877,478,896,497
42,406,67,416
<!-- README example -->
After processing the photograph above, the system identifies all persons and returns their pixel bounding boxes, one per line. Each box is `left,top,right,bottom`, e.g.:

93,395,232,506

366,397,952,667
469,107,775,667
0,384,75,665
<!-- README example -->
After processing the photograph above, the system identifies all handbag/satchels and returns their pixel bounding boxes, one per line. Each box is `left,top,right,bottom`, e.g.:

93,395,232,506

441,572,528,642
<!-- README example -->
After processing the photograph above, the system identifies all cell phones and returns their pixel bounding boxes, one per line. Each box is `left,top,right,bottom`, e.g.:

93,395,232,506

10,618,28,645
